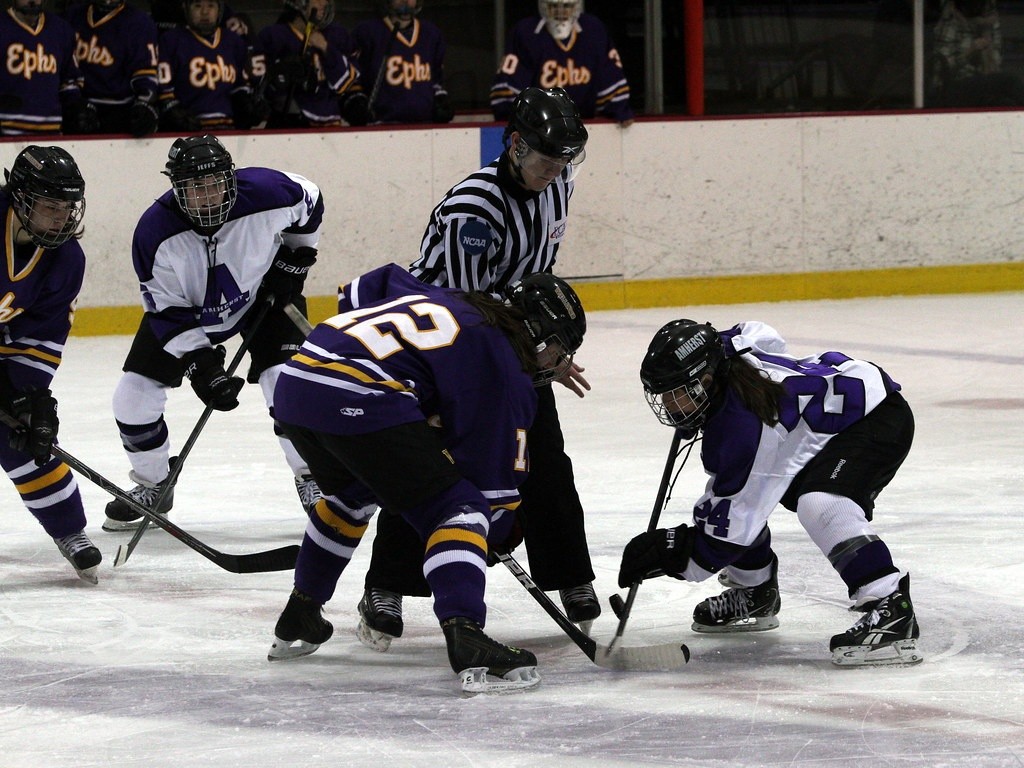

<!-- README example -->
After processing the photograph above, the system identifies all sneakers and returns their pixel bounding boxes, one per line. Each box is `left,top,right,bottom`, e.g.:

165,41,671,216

101,455,181,530
52,530,101,585
442,616,543,693
266,595,334,660
294,472,320,515
691,555,781,634
358,573,404,651
562,580,600,635
827,570,921,666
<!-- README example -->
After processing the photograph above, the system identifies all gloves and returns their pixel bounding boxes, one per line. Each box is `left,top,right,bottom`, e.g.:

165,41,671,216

617,523,692,587
493,101,516,121
178,343,244,412
257,242,317,320
59,89,455,139
488,507,525,570
7,387,60,468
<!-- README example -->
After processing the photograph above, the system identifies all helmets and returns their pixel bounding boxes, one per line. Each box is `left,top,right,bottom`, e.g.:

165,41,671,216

182,0,223,34
161,133,238,228
638,318,717,439
502,85,589,159
283,0,335,31
3,145,85,250
504,271,586,389
538,0,584,40
384,0,423,20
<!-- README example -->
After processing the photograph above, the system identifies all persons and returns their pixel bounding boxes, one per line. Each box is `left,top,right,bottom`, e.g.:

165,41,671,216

1,0,467,129
0,146,107,587
353,87,603,653
98,136,325,530
617,318,923,668
490,1,630,123
268,262,586,695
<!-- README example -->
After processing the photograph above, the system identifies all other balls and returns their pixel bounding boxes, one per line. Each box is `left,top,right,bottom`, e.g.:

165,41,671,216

608,593,629,621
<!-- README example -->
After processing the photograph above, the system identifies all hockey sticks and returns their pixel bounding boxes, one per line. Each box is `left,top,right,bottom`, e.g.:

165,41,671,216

0,410,302,576
111,293,276,570
281,301,692,674
603,427,682,660
